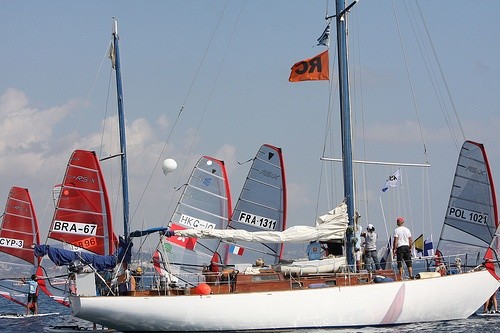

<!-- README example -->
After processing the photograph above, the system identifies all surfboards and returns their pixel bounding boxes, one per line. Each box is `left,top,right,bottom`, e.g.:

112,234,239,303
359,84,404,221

476,312,500,317
0,312,59,319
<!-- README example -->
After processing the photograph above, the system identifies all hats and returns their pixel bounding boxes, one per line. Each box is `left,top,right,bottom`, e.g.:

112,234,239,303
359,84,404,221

366,224,373,229
397,217,403,222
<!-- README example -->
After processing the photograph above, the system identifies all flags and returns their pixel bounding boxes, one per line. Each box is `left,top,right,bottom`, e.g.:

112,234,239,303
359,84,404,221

412,234,424,253
435,251,448,276
380,235,392,270
289,49,329,82
424,235,433,256
382,168,400,193
317,22,331,46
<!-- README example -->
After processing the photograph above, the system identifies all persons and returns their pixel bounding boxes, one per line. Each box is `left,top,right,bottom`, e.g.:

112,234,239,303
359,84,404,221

306,241,328,260
392,217,413,281
354,218,382,272
482,292,500,313
131,267,142,283
22,274,38,315
256,258,264,268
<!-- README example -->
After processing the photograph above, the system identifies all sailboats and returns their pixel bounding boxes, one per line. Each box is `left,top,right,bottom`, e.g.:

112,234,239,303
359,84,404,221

64,0,500,333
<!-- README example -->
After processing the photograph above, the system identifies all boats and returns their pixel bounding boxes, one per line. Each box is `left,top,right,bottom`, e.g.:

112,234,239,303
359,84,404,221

0,277,114,333
